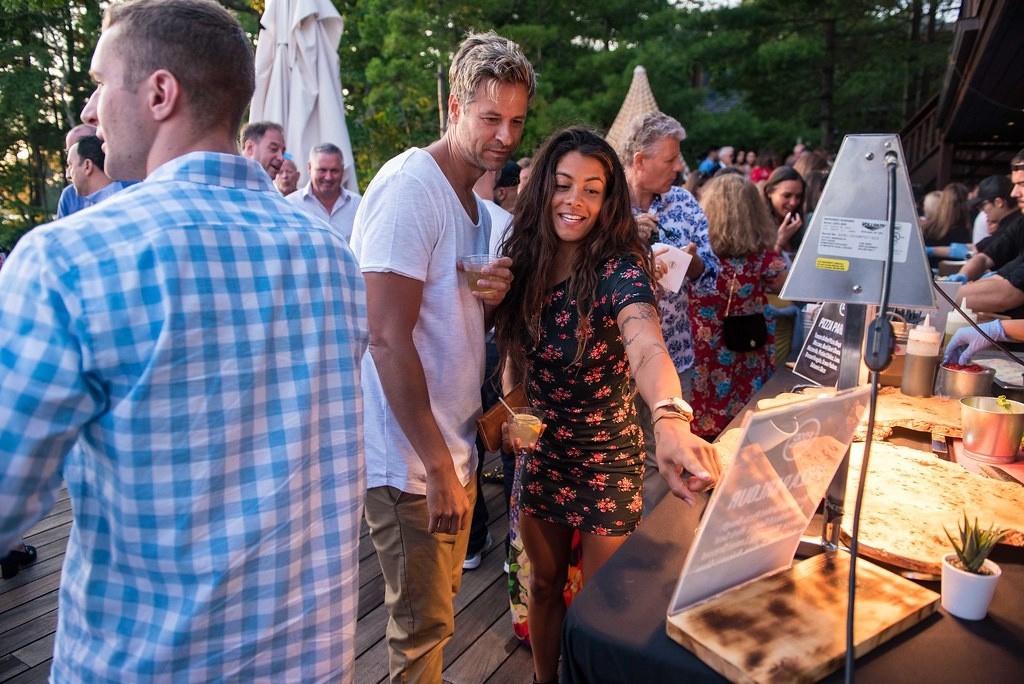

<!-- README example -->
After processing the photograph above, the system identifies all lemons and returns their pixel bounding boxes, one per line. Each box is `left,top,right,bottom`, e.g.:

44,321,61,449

514,413,541,434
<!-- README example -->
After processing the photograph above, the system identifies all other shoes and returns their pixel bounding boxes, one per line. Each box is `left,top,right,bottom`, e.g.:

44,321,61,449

462,533,493,570
483,465,504,483
502,537,510,573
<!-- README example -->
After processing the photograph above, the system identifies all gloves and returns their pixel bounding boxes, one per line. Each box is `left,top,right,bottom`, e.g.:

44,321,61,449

943,318,1006,365
946,273,968,285
948,243,970,258
978,271,998,279
923,245,934,258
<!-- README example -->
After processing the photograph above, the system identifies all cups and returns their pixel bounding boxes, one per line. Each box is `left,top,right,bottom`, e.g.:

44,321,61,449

505,406,545,448
462,254,503,294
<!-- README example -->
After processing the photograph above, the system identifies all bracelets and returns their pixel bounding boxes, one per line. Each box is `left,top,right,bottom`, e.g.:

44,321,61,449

653,411,688,423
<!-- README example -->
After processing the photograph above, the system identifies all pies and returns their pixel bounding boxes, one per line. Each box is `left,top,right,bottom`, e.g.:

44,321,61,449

709,386,1024,575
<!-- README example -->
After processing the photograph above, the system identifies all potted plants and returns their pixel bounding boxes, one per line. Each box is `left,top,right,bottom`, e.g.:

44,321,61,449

940,510,1012,620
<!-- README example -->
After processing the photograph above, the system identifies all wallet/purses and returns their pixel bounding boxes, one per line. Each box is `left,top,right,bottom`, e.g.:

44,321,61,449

477,384,533,453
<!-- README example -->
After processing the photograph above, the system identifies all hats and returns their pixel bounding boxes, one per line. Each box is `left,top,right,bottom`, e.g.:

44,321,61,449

968,176,1013,207
1010,149,1024,171
492,160,522,190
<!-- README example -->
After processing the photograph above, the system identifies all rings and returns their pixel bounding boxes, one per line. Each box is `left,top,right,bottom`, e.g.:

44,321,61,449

655,264,661,271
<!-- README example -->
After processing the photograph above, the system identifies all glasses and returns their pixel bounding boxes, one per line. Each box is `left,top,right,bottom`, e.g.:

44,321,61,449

976,197,1001,211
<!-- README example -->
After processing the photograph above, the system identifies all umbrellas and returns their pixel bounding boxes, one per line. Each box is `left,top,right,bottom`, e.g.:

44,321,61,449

247,0,361,195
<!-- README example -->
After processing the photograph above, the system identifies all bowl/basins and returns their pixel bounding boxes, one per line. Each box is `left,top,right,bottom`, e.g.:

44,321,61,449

959,396,1024,463
940,364,996,399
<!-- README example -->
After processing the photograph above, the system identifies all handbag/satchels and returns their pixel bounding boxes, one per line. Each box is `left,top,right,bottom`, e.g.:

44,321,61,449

724,313,769,351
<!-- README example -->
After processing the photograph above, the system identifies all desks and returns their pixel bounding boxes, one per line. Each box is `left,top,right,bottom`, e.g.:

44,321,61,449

561,343,1024,683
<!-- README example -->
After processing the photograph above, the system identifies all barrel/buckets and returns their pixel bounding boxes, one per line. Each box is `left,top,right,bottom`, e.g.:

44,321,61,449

801,301,822,345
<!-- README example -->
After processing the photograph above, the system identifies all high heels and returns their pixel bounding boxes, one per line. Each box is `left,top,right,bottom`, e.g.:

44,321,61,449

1,543,37,580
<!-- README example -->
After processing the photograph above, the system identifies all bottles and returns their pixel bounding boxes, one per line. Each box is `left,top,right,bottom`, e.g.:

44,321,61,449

942,297,977,356
877,321,914,387
901,314,941,398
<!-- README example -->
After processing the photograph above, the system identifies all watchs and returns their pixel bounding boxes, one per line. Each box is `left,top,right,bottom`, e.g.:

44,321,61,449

651,396,694,422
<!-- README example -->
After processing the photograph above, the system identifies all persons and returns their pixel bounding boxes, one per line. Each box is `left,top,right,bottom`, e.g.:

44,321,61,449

463,155,535,568
55,124,143,218
240,122,287,181
345,30,544,684
0,0,370,683
616,111,720,522
63,136,123,208
495,129,722,683
673,133,838,443
274,158,301,199
912,143,1024,364
283,143,362,246
